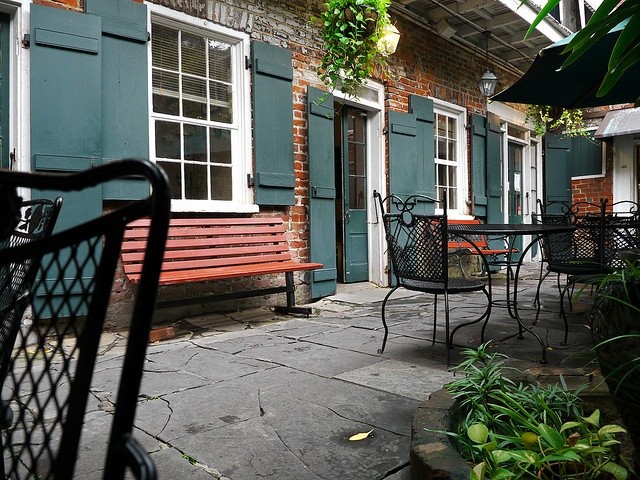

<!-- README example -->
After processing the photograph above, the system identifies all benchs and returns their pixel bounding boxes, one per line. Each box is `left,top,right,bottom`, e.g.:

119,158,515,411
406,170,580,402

118,216,325,341
427,218,520,281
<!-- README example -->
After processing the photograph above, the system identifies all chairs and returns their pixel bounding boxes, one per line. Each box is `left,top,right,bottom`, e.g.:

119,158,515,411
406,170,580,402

1,157,173,478
372,189,490,368
535,197,611,348
2,195,65,394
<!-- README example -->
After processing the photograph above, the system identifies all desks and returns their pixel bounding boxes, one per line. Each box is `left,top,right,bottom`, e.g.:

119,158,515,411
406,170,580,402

447,223,581,366
532,217,640,297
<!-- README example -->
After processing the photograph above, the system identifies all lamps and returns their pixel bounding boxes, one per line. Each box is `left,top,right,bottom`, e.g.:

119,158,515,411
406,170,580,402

477,69,499,98
375,23,401,57
